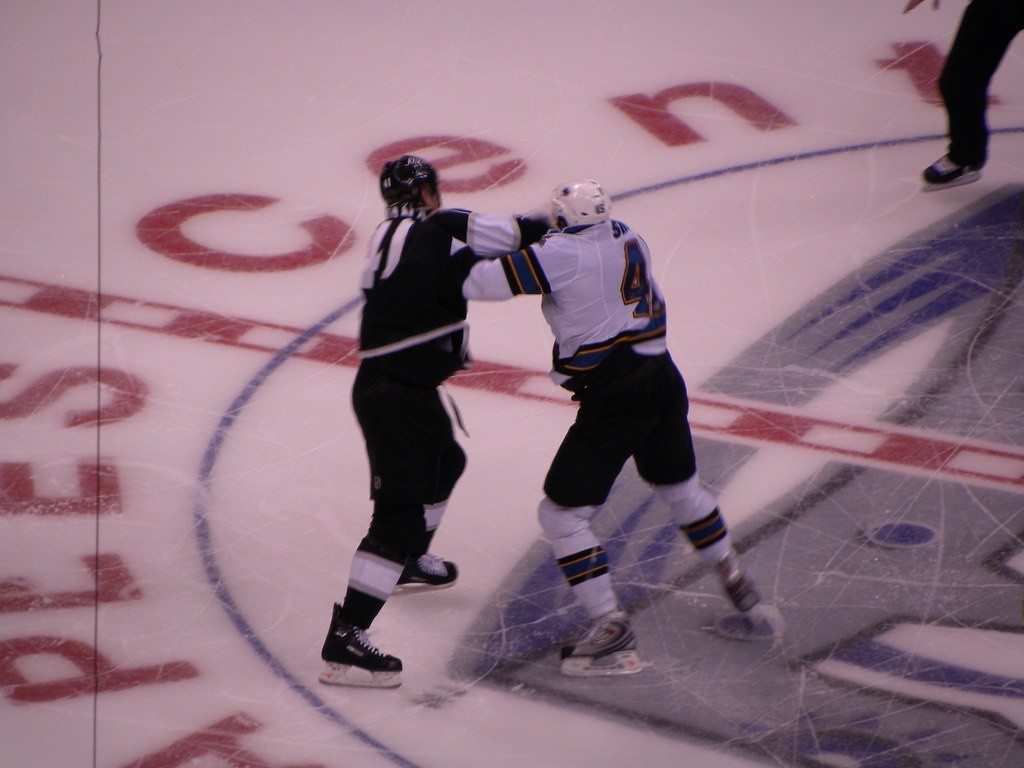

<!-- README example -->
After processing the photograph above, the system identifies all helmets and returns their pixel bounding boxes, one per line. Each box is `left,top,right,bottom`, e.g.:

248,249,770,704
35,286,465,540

379,154,438,206
547,177,610,232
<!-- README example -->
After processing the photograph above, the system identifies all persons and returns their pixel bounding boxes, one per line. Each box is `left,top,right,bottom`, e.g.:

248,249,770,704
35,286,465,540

451,180,782,674
320,150,552,688
902,0,1024,191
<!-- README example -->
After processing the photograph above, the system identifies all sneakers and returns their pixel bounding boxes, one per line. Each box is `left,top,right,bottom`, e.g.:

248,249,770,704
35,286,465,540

392,554,458,596
559,609,643,677
320,603,403,688
715,604,786,641
921,155,988,191
